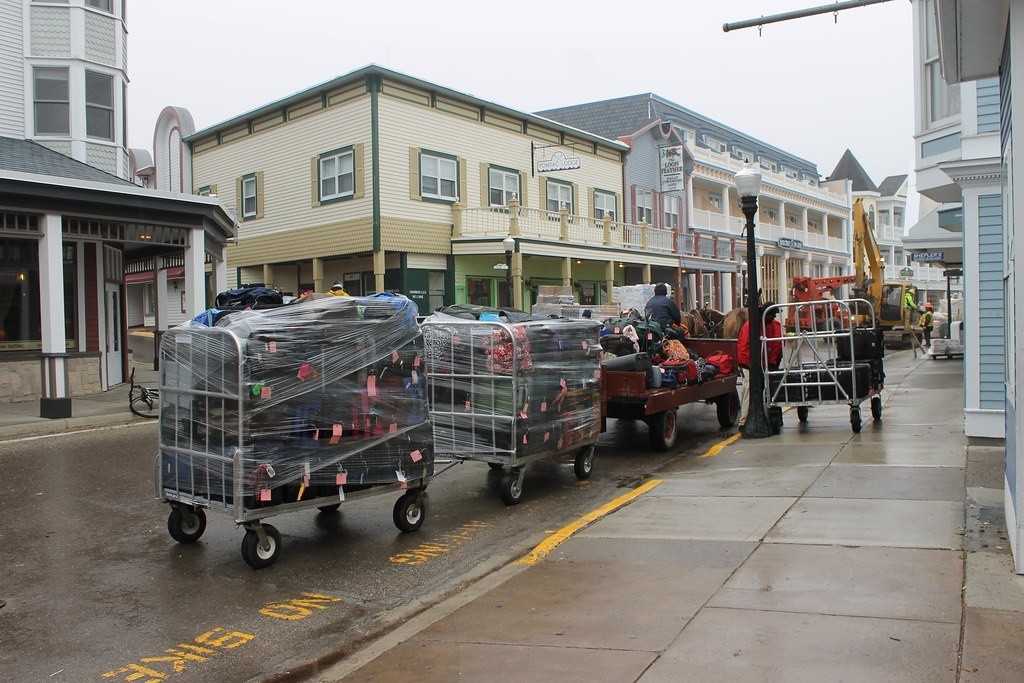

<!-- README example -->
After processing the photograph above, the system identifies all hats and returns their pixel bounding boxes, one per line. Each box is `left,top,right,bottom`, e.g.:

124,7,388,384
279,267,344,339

925,302,933,307
759,302,783,313
655,284,667,296
908,289,915,295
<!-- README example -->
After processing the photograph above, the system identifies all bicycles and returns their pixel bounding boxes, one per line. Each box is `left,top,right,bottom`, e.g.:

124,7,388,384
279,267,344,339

128,363,162,420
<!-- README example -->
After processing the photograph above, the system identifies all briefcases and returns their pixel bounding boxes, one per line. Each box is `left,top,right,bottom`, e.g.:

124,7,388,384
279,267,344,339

768,327,885,399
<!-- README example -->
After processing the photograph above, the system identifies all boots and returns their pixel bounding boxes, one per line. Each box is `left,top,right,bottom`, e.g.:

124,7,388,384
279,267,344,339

923,339,931,347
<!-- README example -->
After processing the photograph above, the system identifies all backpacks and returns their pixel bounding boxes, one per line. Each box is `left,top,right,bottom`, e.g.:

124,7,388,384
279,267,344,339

599,308,732,387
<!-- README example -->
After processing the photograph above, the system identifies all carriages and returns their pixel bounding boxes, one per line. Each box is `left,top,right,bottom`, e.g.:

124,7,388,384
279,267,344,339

604,298,771,450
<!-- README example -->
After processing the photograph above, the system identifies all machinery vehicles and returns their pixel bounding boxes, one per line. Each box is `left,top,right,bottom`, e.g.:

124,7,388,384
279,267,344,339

850,196,925,352
782,272,869,343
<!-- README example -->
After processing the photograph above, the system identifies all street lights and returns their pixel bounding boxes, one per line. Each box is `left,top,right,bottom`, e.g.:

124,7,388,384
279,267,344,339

736,167,770,443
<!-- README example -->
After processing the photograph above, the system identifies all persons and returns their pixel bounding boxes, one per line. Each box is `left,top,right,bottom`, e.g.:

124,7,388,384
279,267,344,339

645,283,681,328
918,302,933,348
737,302,782,432
903,288,918,317
